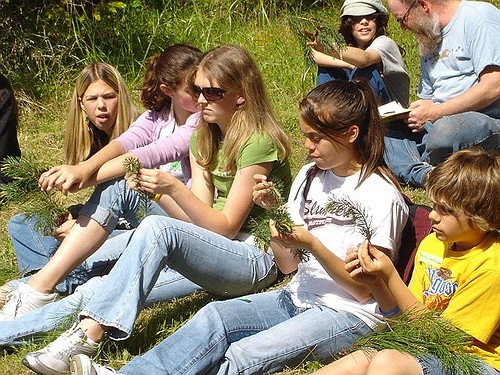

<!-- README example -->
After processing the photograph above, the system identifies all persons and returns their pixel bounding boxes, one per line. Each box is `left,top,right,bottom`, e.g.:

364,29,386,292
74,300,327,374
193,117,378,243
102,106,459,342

301,0,410,108
307,147,500,375
70,80,409,375
9,63,174,297
0,45,294,375
382,0,500,190
0,43,203,324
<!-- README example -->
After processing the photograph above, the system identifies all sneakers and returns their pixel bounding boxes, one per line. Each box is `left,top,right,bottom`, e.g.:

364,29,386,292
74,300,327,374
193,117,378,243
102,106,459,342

22,322,102,375
0,283,59,321
70,354,116,375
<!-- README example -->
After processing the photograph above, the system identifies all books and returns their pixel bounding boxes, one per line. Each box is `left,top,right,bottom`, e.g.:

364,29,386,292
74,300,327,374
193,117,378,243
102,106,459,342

377,101,411,124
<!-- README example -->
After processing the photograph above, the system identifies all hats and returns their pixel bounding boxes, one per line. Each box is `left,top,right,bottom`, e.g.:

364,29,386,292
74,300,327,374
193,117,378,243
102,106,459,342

339,0,388,18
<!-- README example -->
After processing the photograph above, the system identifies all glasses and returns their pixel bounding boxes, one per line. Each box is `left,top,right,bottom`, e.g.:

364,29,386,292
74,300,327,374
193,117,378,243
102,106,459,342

192,82,226,101
399,0,416,28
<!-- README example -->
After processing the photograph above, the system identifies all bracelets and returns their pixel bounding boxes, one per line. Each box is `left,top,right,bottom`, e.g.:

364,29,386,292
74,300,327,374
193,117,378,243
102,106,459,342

152,194,162,201
378,306,399,317
